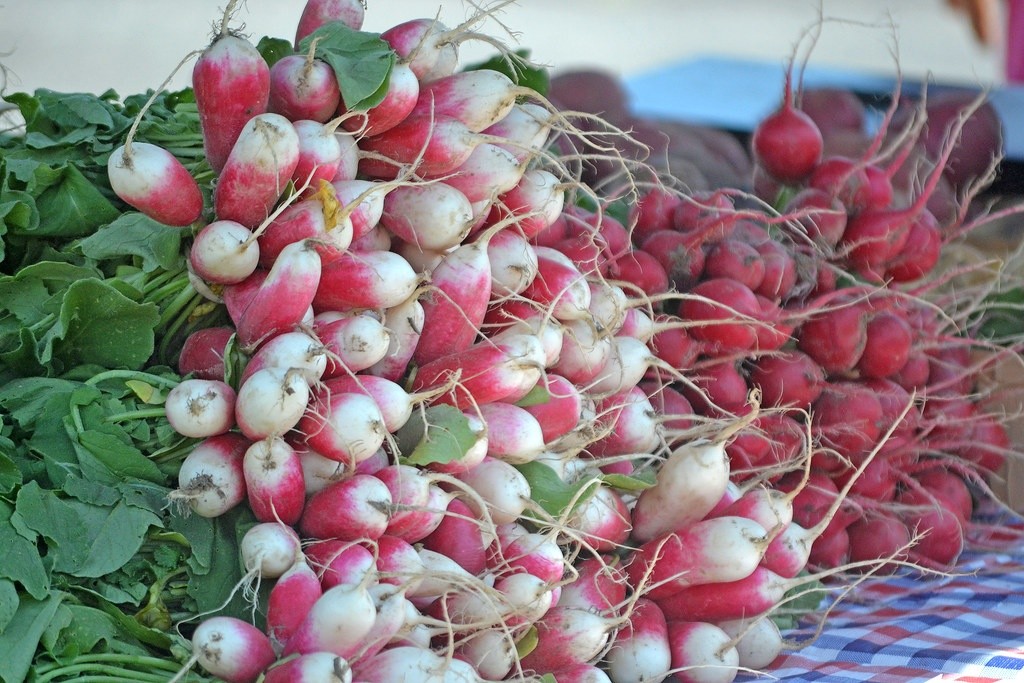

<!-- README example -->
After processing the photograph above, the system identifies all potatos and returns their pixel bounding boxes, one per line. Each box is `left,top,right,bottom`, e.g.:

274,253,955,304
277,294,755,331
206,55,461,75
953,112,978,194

543,70,1003,228
